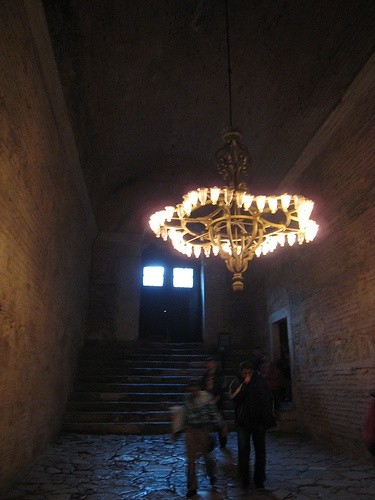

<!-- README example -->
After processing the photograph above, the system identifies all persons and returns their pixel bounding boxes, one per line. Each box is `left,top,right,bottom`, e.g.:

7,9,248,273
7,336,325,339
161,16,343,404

228,360,273,486
198,356,228,450
170,375,228,498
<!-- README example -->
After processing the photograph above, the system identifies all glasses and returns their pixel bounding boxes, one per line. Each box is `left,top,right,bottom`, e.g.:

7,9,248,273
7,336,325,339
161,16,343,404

243,370,253,377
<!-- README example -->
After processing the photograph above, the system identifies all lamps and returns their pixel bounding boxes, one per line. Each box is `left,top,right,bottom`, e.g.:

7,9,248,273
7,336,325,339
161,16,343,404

145,127,319,293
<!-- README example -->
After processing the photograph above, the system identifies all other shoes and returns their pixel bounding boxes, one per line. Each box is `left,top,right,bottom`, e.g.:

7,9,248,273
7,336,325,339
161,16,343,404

241,480,265,490
